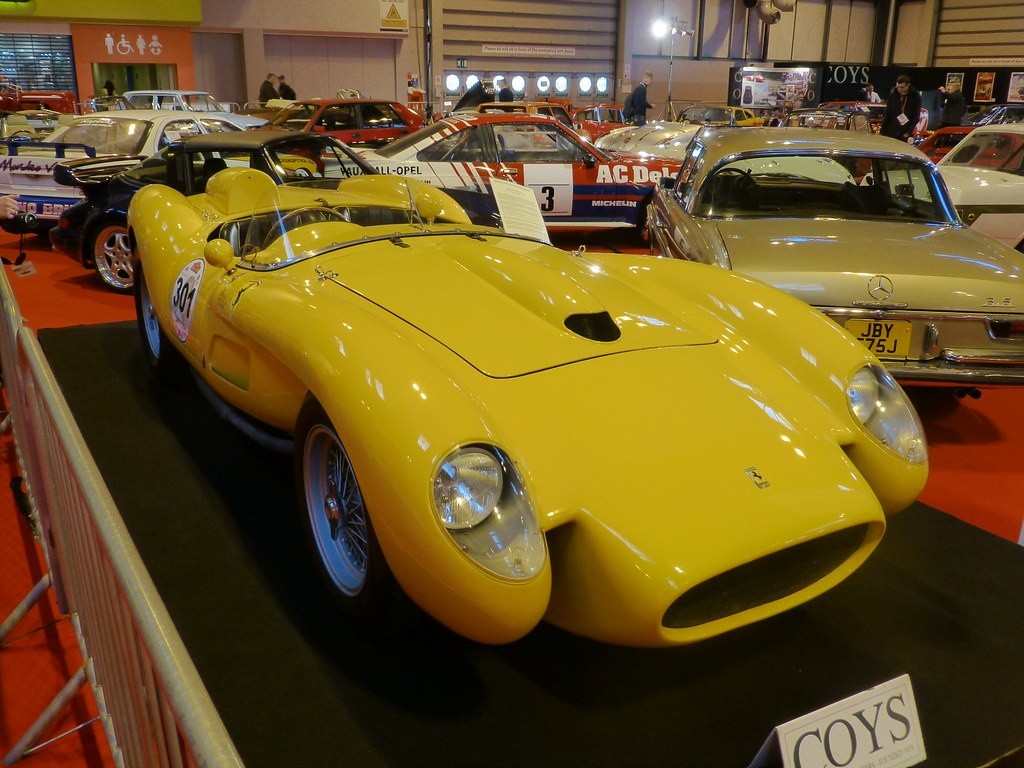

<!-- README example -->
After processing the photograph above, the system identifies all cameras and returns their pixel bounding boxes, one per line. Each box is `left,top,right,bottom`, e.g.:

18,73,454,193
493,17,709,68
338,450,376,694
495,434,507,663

0,209,39,233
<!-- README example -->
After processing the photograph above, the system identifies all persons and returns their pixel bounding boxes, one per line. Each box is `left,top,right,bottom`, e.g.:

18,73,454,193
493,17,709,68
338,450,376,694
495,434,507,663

259,73,279,107
630,72,655,126
938,83,966,127
879,74,921,144
100,80,117,111
0,194,20,219
498,79,514,112
276,75,295,100
855,82,880,103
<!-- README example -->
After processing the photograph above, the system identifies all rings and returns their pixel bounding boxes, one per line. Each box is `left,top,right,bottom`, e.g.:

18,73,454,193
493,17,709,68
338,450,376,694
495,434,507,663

15,211,18,215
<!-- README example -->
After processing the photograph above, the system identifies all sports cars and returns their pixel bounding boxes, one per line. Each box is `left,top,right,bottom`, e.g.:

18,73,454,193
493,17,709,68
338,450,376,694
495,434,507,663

125,166,928,661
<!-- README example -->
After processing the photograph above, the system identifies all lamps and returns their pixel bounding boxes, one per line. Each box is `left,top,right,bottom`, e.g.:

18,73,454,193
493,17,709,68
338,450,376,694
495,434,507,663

669,25,676,34
678,28,686,37
684,28,695,36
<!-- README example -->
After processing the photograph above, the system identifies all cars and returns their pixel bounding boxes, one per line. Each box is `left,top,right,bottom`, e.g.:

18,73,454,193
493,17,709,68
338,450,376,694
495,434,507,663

0,76,1024,398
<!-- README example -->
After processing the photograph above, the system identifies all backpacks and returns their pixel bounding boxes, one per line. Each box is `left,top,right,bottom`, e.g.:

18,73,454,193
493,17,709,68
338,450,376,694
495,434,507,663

622,87,646,119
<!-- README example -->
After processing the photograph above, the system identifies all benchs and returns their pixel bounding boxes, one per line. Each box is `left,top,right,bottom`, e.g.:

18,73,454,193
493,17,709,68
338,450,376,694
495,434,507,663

219,205,408,256
754,186,845,206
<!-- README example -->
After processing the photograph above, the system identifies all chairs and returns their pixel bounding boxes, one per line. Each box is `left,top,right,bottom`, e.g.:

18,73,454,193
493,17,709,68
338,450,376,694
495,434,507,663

202,159,226,180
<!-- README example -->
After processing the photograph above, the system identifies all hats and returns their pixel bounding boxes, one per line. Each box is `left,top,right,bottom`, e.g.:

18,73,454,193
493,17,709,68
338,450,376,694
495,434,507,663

277,75,285,79
